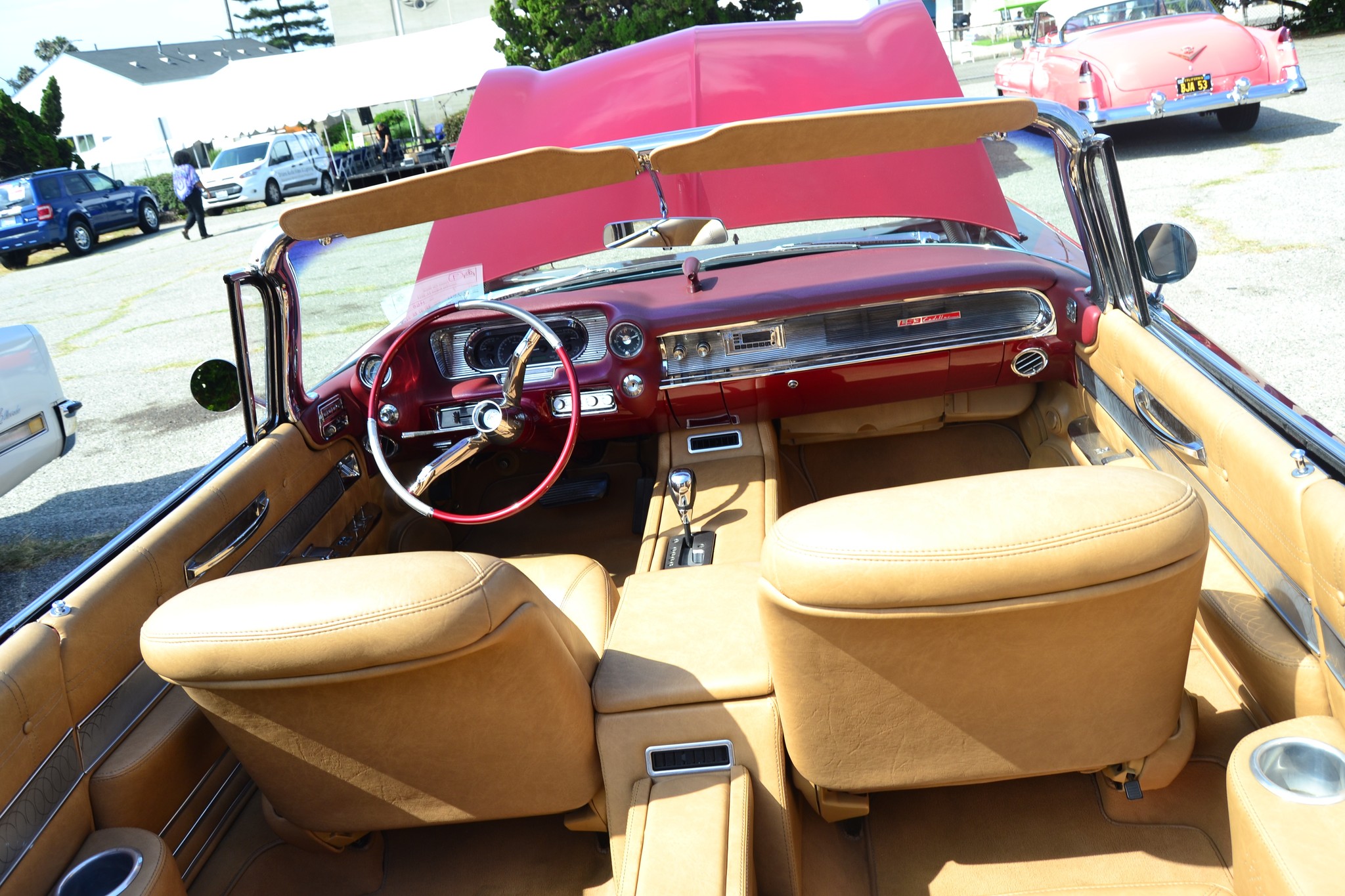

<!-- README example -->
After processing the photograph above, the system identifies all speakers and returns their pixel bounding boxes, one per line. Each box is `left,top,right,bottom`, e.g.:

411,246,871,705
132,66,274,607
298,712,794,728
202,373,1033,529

356,106,375,125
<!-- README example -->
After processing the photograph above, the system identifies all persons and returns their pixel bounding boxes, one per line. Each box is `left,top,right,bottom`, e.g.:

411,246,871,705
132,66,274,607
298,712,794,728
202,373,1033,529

375,122,395,168
956,13,971,41
172,151,213,239
1014,12,1031,38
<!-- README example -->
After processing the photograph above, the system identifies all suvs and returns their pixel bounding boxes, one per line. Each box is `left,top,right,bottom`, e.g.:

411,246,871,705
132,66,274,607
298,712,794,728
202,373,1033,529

0,168,163,271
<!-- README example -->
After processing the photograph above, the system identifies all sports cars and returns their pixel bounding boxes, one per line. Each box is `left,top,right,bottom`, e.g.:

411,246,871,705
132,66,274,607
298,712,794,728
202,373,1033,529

0,0,1345,896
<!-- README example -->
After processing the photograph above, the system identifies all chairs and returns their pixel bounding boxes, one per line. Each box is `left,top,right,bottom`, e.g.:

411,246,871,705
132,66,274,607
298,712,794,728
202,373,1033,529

759,465,1210,824
139,553,620,844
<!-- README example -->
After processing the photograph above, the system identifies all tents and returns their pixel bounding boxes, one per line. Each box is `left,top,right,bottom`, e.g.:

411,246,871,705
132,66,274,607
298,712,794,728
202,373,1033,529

165,8,550,177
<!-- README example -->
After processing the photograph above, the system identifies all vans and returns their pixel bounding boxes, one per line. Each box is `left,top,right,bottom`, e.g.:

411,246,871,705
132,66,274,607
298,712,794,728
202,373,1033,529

200,131,334,216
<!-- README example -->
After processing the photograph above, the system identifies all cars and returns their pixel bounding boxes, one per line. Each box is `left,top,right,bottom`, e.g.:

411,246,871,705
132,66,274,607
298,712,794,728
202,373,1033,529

0,322,83,504
995,1,1309,137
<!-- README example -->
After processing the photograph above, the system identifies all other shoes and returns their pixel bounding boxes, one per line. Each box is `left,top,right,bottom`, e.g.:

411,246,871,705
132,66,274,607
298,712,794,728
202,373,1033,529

202,234,213,239
182,230,190,240
